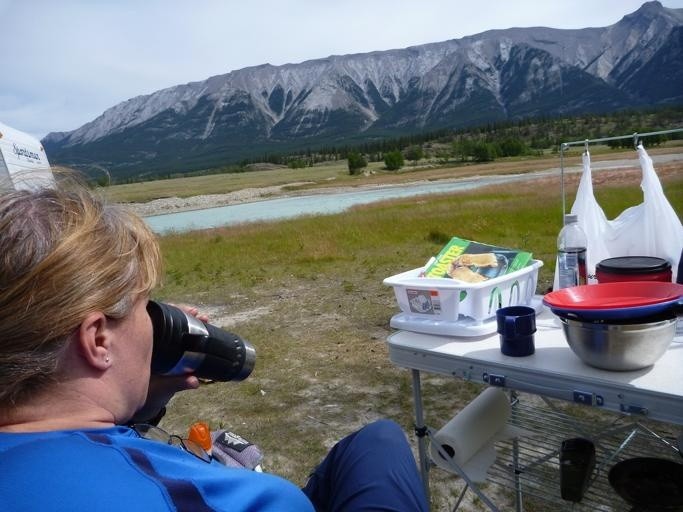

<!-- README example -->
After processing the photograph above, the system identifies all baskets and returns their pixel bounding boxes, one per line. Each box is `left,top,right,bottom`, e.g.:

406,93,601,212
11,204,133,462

382,257,543,322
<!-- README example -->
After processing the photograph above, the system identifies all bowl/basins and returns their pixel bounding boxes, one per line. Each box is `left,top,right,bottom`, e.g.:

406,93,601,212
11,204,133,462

606,456,681,510
558,315,680,373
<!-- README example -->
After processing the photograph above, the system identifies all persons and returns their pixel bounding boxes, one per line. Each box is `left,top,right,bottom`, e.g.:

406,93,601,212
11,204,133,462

0,165,429,512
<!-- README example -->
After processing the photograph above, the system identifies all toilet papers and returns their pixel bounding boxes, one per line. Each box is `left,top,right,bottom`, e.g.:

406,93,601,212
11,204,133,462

428,385,528,485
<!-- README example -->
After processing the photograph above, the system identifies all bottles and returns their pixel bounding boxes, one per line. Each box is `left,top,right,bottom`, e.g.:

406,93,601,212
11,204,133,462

556,212,590,289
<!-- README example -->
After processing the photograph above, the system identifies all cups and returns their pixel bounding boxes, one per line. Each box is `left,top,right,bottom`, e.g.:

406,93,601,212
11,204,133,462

146,301,257,382
495,304,536,358
556,437,597,503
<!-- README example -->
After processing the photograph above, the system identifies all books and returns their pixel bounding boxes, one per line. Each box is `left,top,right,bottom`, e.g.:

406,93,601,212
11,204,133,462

422,237,532,322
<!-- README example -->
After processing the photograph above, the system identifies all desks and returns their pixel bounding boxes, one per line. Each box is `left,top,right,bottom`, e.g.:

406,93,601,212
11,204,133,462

385,295,683,512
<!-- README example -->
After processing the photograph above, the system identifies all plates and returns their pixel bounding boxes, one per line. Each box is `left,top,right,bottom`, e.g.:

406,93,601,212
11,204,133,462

543,281,683,321
